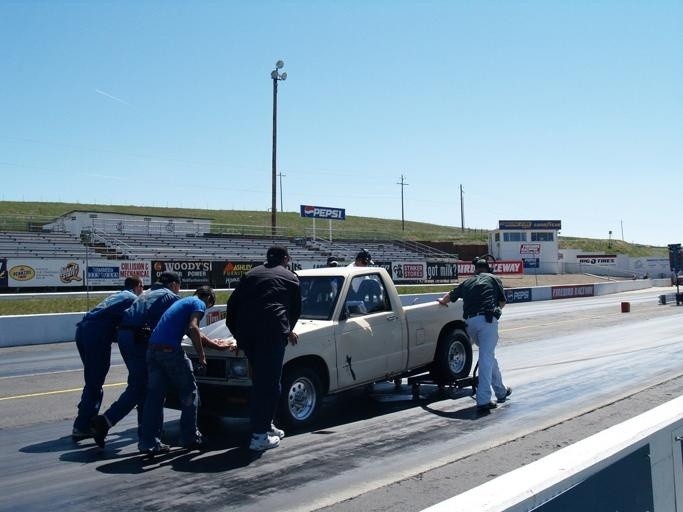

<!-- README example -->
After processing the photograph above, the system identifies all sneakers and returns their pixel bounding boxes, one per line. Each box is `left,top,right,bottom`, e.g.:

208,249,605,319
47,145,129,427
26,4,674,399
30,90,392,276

71,425,96,437
267,420,286,438
138,438,171,452
89,415,109,448
249,431,281,452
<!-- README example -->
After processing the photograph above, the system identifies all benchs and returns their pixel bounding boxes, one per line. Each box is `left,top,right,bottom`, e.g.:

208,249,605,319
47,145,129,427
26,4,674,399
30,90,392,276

305,241,431,262
0,230,101,258
91,232,328,258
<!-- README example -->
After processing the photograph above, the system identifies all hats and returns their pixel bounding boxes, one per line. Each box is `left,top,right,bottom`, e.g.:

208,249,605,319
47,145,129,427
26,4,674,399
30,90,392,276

359,249,375,265
327,256,338,265
475,258,489,268
267,245,291,259
152,270,180,286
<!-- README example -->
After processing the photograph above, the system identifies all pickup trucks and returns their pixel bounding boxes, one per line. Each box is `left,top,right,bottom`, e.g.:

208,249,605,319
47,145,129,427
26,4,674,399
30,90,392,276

159,267,472,429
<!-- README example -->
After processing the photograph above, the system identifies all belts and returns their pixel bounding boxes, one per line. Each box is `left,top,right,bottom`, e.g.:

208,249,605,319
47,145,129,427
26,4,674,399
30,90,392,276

464,312,486,318
145,344,173,353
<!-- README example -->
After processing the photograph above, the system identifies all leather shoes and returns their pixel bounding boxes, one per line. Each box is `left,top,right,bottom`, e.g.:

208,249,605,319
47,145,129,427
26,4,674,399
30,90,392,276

497,387,512,403
478,401,497,410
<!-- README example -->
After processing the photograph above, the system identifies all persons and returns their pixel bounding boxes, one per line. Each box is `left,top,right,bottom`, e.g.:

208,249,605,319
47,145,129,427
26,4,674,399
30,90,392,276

345,250,374,295
306,256,355,304
435,259,511,409
138,285,230,455
225,243,301,452
70,277,144,440
90,272,223,449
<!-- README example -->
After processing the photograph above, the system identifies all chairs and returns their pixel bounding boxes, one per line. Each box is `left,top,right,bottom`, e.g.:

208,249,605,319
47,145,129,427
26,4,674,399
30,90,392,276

307,280,333,314
357,279,383,311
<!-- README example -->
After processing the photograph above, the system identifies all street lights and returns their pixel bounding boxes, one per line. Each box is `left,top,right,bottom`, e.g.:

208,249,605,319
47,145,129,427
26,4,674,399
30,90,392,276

270,58,288,236
